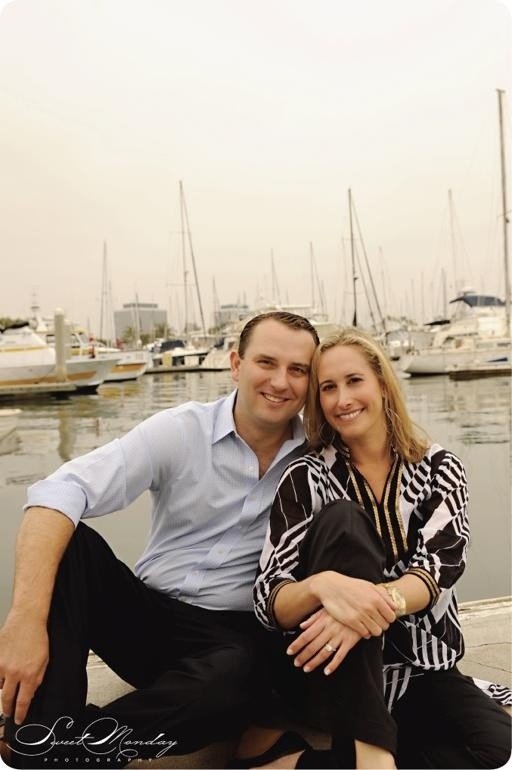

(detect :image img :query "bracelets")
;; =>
[381,581,407,618]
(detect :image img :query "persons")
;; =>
[251,324,512,769]
[0,308,309,769]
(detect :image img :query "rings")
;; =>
[324,643,337,653]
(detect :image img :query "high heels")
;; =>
[224,726,311,769]
